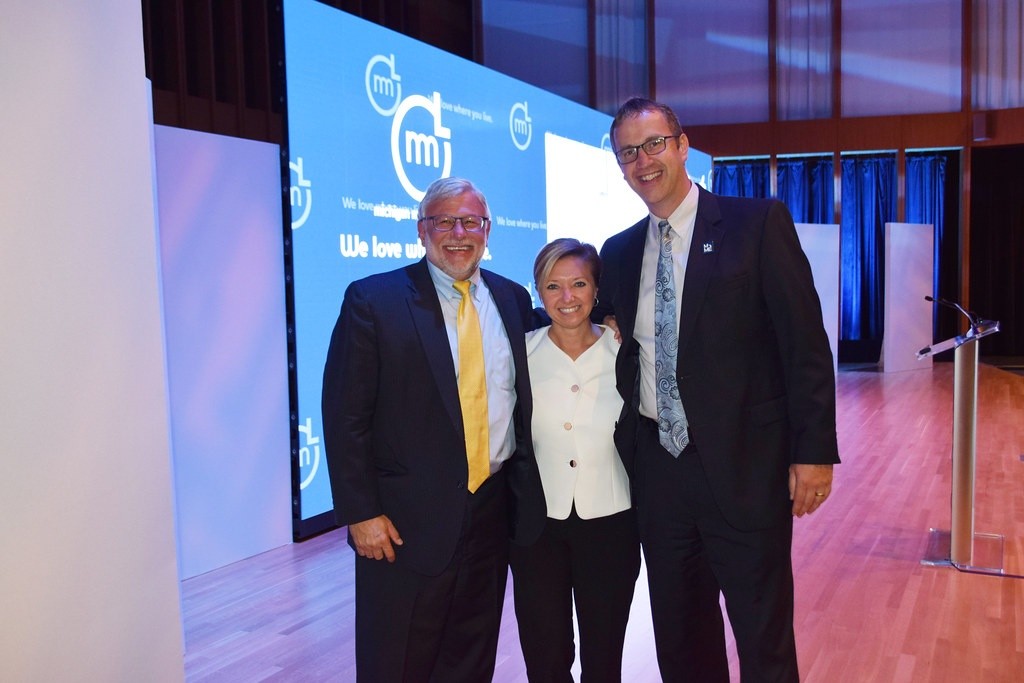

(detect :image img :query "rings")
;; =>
[817,493,824,496]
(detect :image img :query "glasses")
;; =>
[615,135,680,164]
[417,214,489,231]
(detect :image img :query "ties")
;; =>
[451,280,490,494]
[655,221,690,458]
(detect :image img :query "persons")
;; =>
[509,238,645,683]
[598,97,841,682]
[320,178,622,683]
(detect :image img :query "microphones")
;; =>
[943,297,974,327]
[925,295,979,322]
